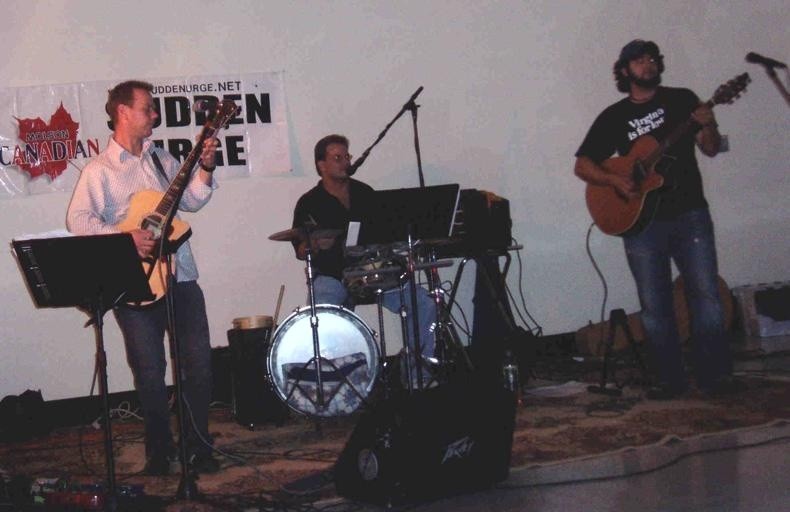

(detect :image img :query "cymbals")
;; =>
[268,224,343,242]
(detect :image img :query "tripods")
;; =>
[284,249,366,417]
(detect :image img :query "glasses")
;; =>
[329,153,353,161]
[634,54,657,64]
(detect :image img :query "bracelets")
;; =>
[198,159,217,173]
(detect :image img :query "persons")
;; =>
[66,80,222,477]
[286,134,442,393]
[573,39,744,400]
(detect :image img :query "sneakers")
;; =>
[140,438,221,479]
[644,369,750,398]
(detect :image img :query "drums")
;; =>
[264,304,380,419]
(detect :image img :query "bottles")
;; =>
[502,350,519,393]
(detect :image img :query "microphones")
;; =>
[744,52,786,69]
[192,99,219,112]
[347,157,364,176]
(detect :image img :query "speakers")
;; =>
[332,380,518,509]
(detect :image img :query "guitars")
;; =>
[115,99,240,311]
[585,73,751,237]
